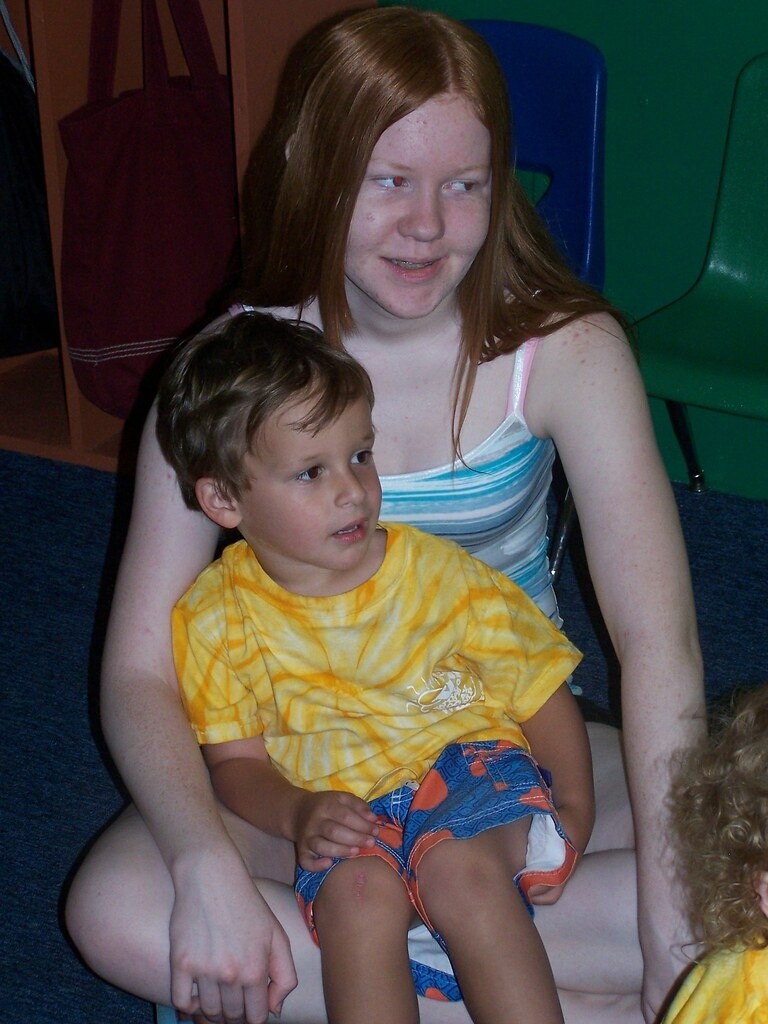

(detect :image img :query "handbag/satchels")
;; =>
[60,2,242,420]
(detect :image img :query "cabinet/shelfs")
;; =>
[0,0,378,480]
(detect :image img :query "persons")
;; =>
[67,4,723,1024]
[153,308,596,1024]
[656,690,768,1024]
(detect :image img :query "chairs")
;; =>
[608,51,767,501]
[432,18,615,324]
